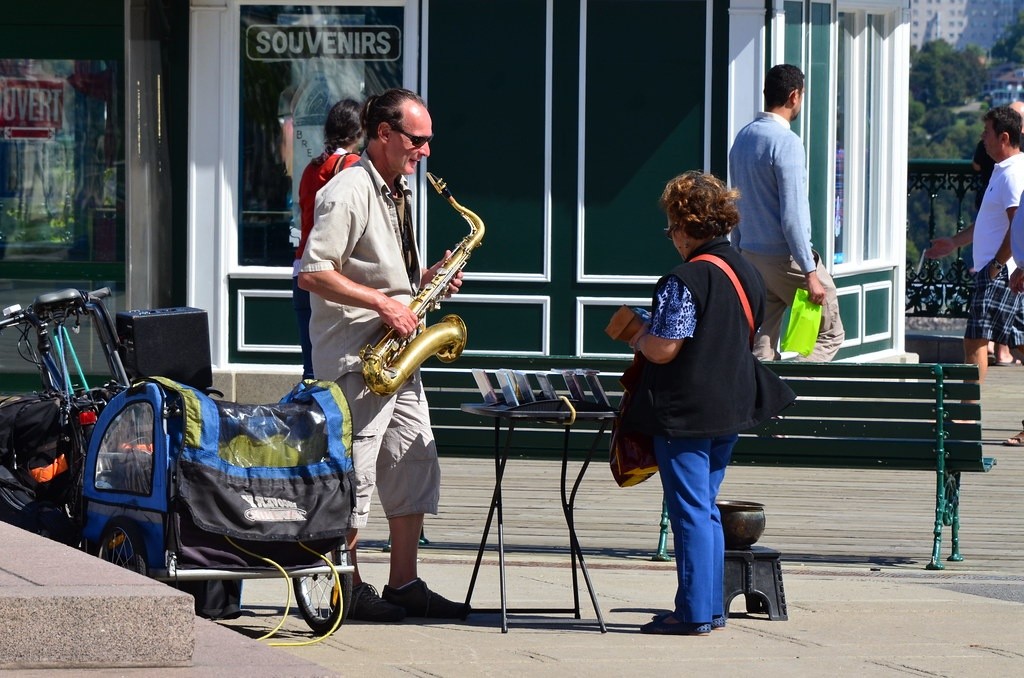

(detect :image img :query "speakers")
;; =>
[117,306,213,390]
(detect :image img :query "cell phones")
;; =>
[562,372,587,402]
[535,371,559,400]
[496,367,537,407]
[583,373,611,407]
[471,369,498,403]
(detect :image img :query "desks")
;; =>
[458,400,614,633]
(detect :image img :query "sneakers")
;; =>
[383,579,471,618]
[328,582,407,622]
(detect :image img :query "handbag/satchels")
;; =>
[609,347,662,487]
[774,287,822,362]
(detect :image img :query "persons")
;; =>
[729,65,845,363]
[627,170,797,636]
[924,101,1024,447]
[293,99,363,381]
[297,88,473,625]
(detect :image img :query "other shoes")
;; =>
[641,617,711,636]
[654,613,726,630]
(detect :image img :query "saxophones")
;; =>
[359,172,486,396]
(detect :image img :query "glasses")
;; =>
[662,220,683,237]
[392,129,436,145]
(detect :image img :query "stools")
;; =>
[675,544,789,622]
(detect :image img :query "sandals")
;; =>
[1003,431,1024,446]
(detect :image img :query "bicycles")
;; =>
[0,285,114,517]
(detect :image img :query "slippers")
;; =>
[987,353,1022,367]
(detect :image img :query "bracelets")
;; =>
[993,259,1004,273]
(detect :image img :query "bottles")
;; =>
[290,56,343,231]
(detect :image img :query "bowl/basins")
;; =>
[714,499,766,550]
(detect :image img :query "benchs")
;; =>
[420,354,999,570]
